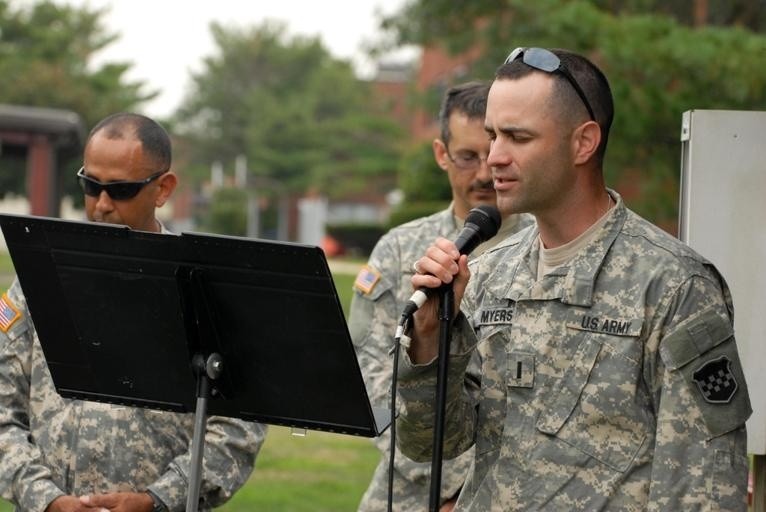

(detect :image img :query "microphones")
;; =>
[401,205,502,316]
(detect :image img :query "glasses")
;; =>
[76,159,172,199]
[504,42,601,128]
[441,141,492,172]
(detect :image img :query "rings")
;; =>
[410,259,426,275]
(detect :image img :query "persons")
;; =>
[347,79,538,512]
[387,44,755,511]
[0,110,269,512]
